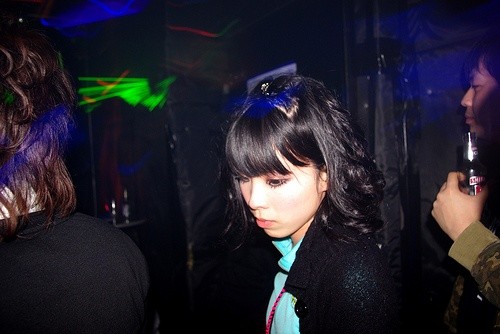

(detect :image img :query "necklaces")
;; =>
[265,286,286,334]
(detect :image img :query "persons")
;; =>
[0,22,161,334]
[431,43,500,334]
[225,73,393,334]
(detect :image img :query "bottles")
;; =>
[460,132,485,195]
